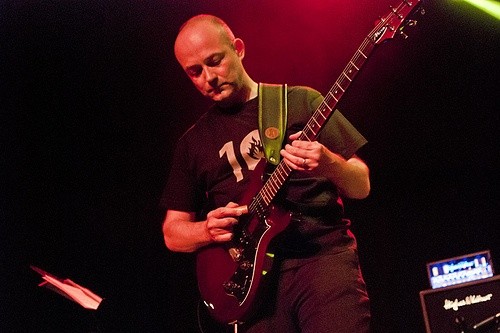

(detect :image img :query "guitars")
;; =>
[196,0,425,326]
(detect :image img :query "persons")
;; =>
[159,13,370,333]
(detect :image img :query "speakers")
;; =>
[419,274,500,333]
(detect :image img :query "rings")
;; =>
[302,159,306,168]
